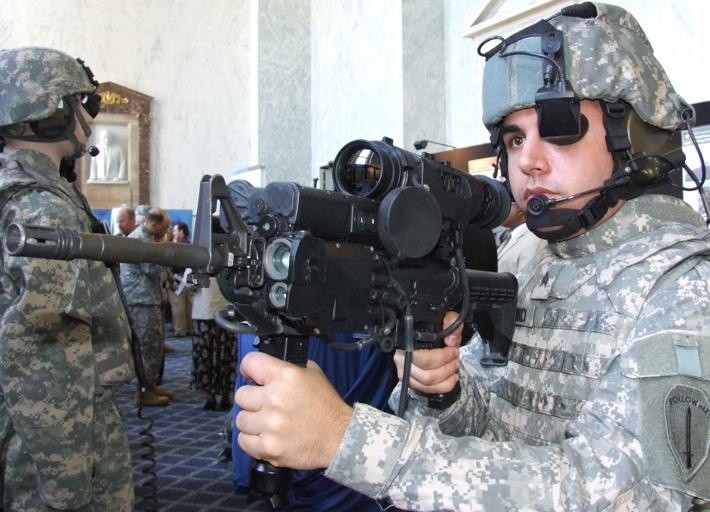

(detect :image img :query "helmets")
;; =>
[481,1,695,144]
[0,48,95,124]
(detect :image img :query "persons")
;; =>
[167,222,191,338]
[234,1,710,512]
[119,206,173,406]
[111,208,138,283]
[134,205,152,227]
[0,46,135,512]
[187,215,234,412]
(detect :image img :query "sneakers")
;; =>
[137,390,168,407]
[153,386,173,398]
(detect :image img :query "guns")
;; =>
[0,138,519,501]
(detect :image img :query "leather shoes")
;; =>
[216,395,232,410]
[193,394,216,411]
